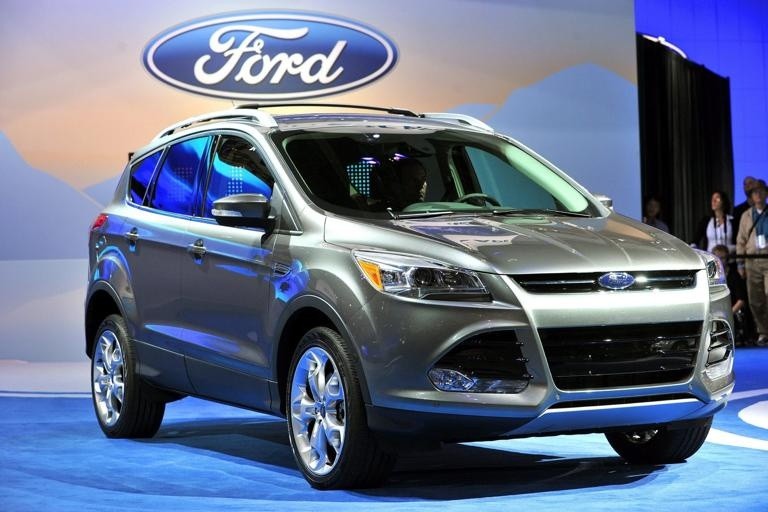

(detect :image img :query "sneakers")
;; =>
[756,334,767,345]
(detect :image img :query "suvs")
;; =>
[82,101,736,493]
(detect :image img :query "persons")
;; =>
[699,192,740,263]
[730,176,757,246]
[637,195,675,233]
[369,152,431,213]
[700,240,749,337]
[744,176,768,343]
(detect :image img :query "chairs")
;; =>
[316,160,399,208]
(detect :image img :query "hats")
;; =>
[745,180,768,197]
